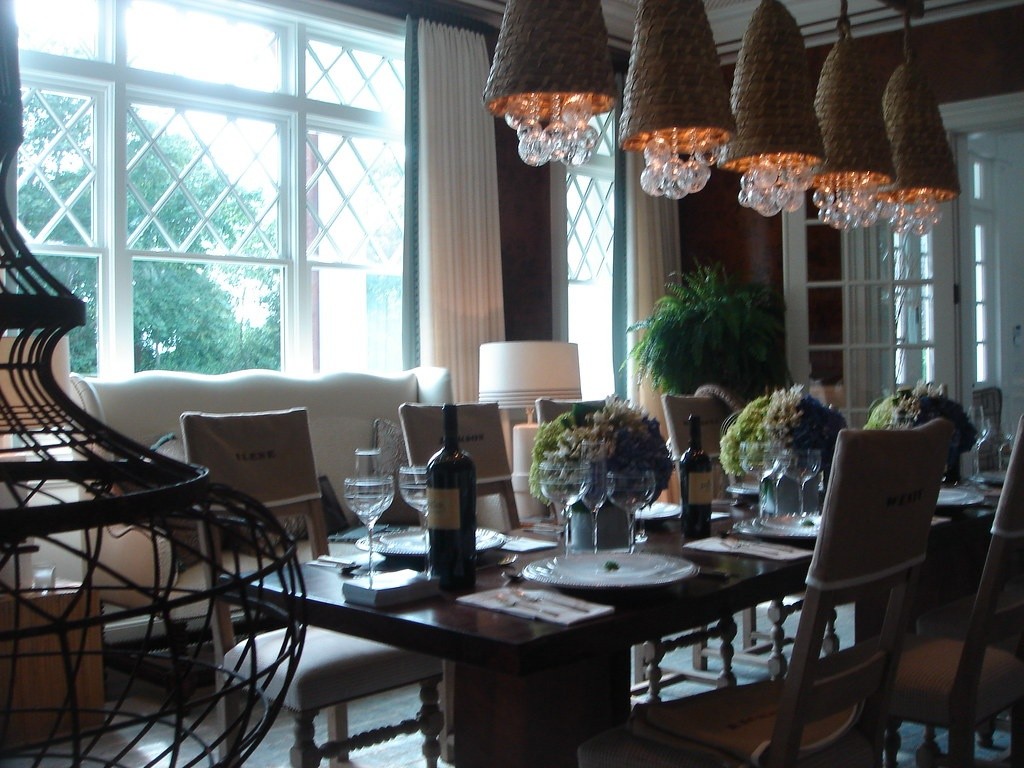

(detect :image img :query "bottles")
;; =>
[426,405,477,591]
[679,415,712,538]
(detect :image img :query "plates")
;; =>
[733,515,822,537]
[636,502,682,519]
[356,528,507,564]
[522,551,700,587]
[936,489,983,505]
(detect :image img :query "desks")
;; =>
[210,477,997,768]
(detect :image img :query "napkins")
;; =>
[340,567,441,608]
[454,581,615,627]
[682,535,813,564]
[500,535,559,553]
[929,514,953,527]
[676,511,731,522]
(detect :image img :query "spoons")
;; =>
[497,593,558,617]
[513,591,588,613]
[305,561,362,572]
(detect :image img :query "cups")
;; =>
[33,562,54,595]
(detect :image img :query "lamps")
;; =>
[477,341,584,493]
[479,0,961,237]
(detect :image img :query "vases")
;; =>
[757,474,820,515]
[569,505,634,551]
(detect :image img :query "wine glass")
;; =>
[783,450,822,526]
[345,448,395,588]
[986,416,1014,478]
[740,442,781,527]
[540,461,591,566]
[967,406,990,483]
[607,472,656,563]
[581,441,607,571]
[768,448,792,527]
[399,467,439,583]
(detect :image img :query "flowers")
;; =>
[862,378,979,455]
[528,392,676,512]
[719,383,849,501]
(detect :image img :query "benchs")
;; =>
[30,365,456,718]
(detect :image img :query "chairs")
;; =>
[178,407,446,768]
[536,393,738,703]
[816,413,1024,768]
[577,415,949,768]
[397,401,521,530]
[659,392,840,682]
